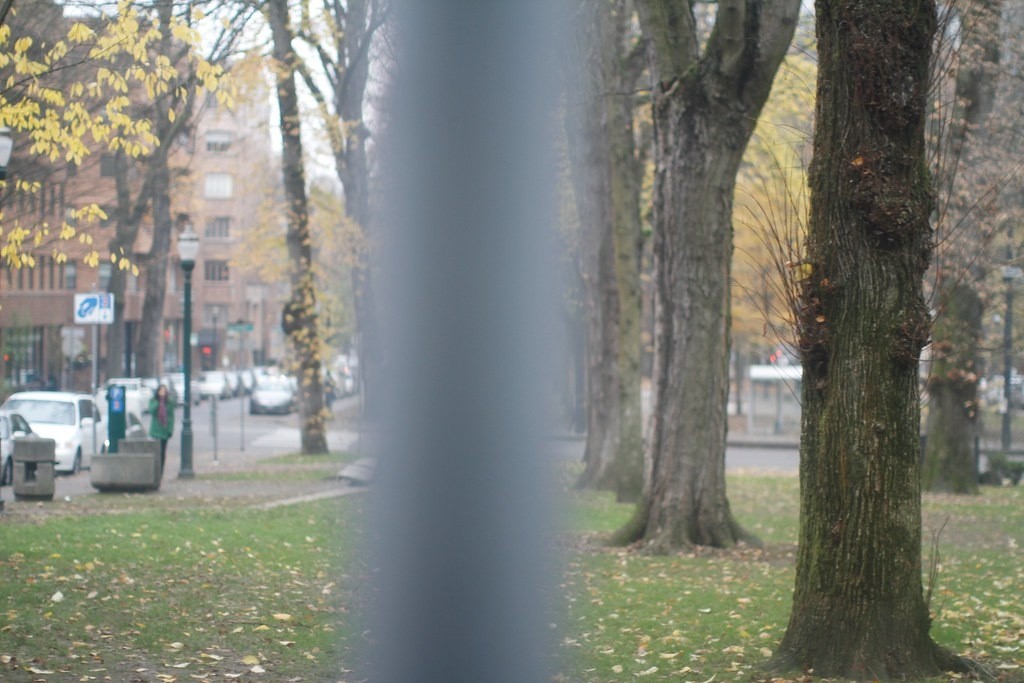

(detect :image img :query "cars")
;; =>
[248,375,293,415]
[320,355,361,401]
[0,411,41,486]
[90,367,278,433]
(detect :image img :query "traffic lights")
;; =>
[201,343,213,372]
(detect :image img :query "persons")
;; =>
[149,385,175,476]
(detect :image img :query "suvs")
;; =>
[2,390,107,477]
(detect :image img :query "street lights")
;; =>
[173,223,200,481]
